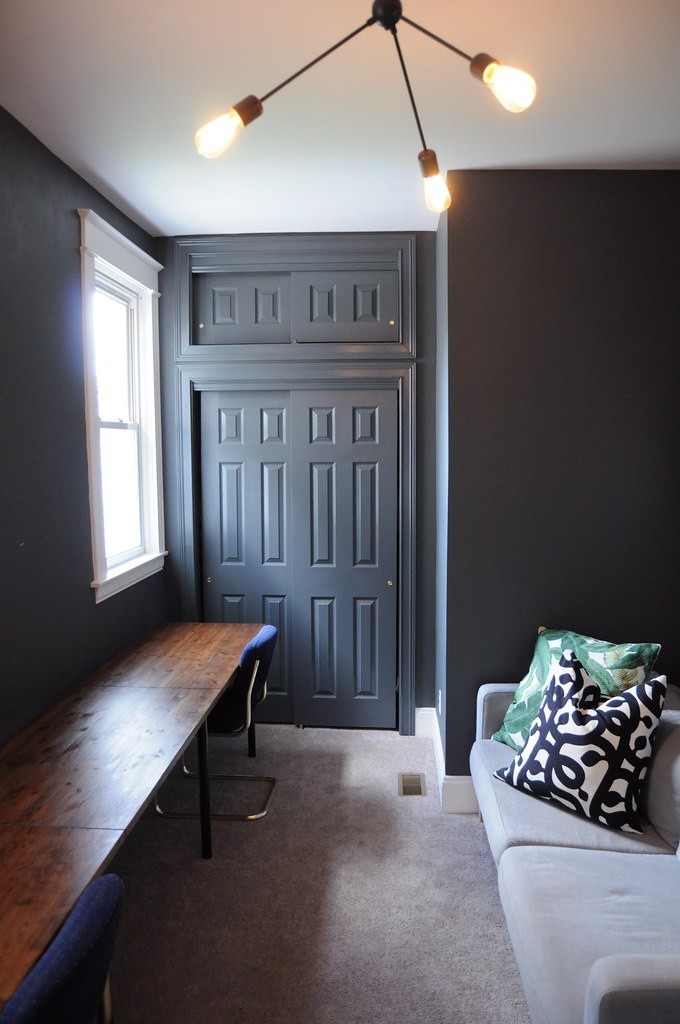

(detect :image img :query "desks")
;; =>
[0,621,268,1024]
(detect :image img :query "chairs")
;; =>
[0,871,129,1024]
[154,624,279,823]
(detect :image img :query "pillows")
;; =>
[490,627,662,755]
[492,649,668,836]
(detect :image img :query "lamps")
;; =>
[192,0,539,214]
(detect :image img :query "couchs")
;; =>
[469,682,680,1024]
